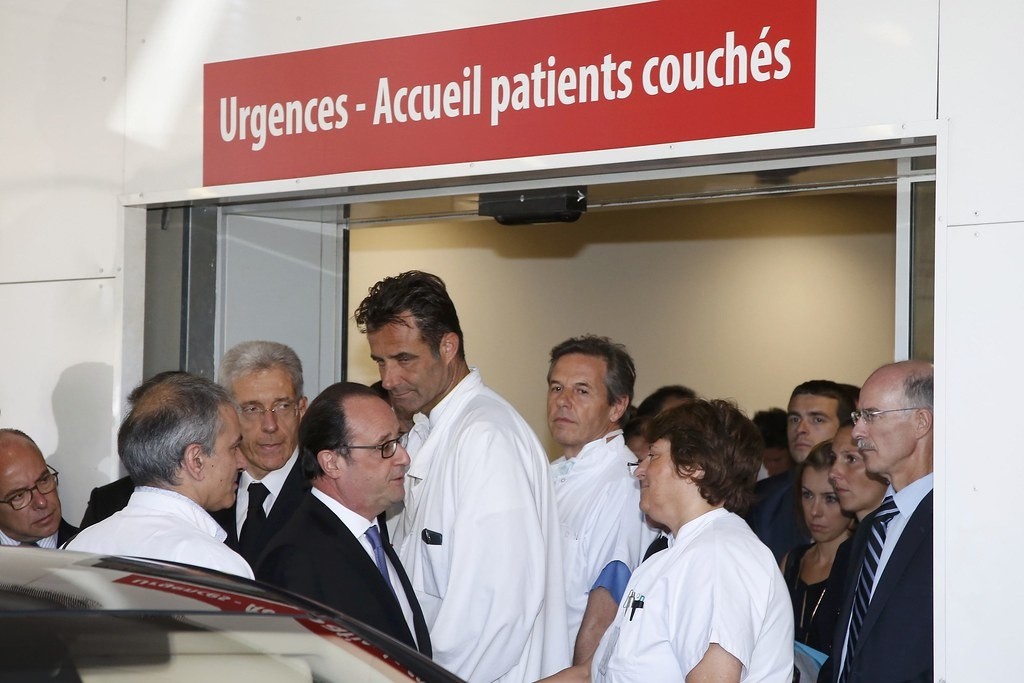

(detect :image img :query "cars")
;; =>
[0,545,470,683]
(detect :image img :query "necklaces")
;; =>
[797,547,830,643]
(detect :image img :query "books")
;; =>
[793,640,830,683]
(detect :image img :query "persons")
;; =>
[821,394,890,683]
[538,399,795,683]
[210,339,308,572]
[740,379,857,611]
[787,438,859,682]
[367,380,415,562]
[830,355,933,683]
[546,330,662,683]
[612,380,868,482]
[80,371,193,531]
[355,270,567,682]
[255,383,433,661]
[57,374,256,582]
[0,428,84,551]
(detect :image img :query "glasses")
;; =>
[850,407,920,426]
[238,400,299,422]
[332,430,409,458]
[0,464,58,510]
[627,461,642,479]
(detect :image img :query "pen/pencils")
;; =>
[622,589,644,621]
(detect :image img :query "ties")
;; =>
[238,481,271,562]
[363,525,401,610]
[838,494,900,683]
